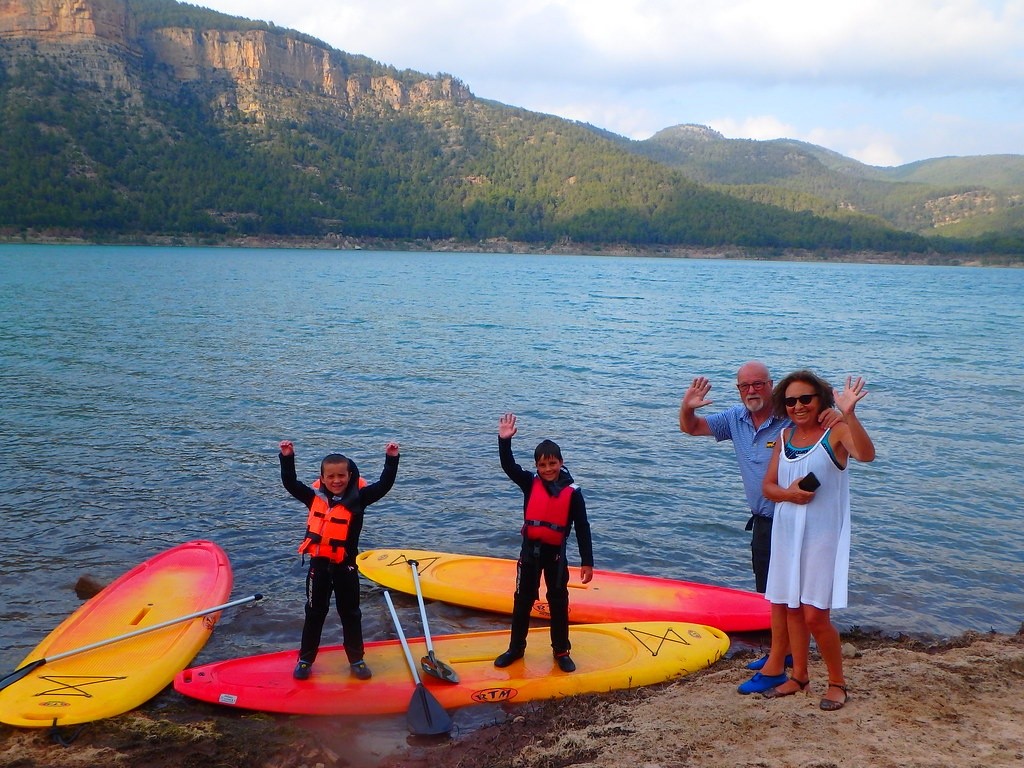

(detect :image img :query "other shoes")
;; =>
[350,660,372,680]
[747,652,793,669]
[494,649,524,668]
[293,660,312,678]
[737,671,788,694]
[552,648,576,673]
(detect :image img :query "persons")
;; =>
[493,412,594,673]
[763,370,875,711]
[679,362,847,694]
[278,439,401,680]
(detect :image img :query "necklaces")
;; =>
[800,429,817,440]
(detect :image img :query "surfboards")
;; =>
[355,545,782,633]
[0,538,235,730]
[175,620,737,719]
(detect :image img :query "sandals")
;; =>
[819,684,847,711]
[762,677,810,699]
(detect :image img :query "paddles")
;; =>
[407,558,458,681]
[377,589,455,738]
[0,595,267,690]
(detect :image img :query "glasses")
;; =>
[784,393,819,407]
[737,380,773,392]
